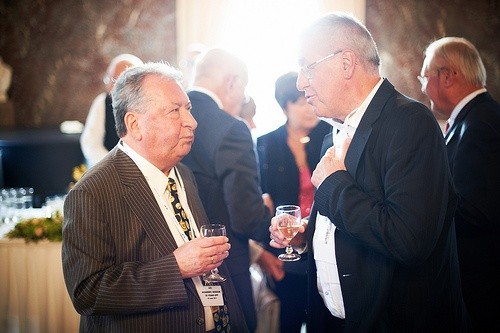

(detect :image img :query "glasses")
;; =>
[302,50,342,78]
[417,69,457,84]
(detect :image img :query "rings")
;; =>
[210,255,213,264]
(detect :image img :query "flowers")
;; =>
[4,210,63,243]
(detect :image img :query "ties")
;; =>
[445,121,449,132]
[165,177,231,333]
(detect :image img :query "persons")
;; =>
[269,15,448,332]
[173,48,273,332]
[78,53,145,167]
[239,94,258,130]
[62,65,231,333]
[256,71,342,333]
[417,36,499,332]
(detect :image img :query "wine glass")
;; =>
[275,204,301,261]
[200,224,227,282]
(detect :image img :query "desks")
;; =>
[0,232,81,333]
